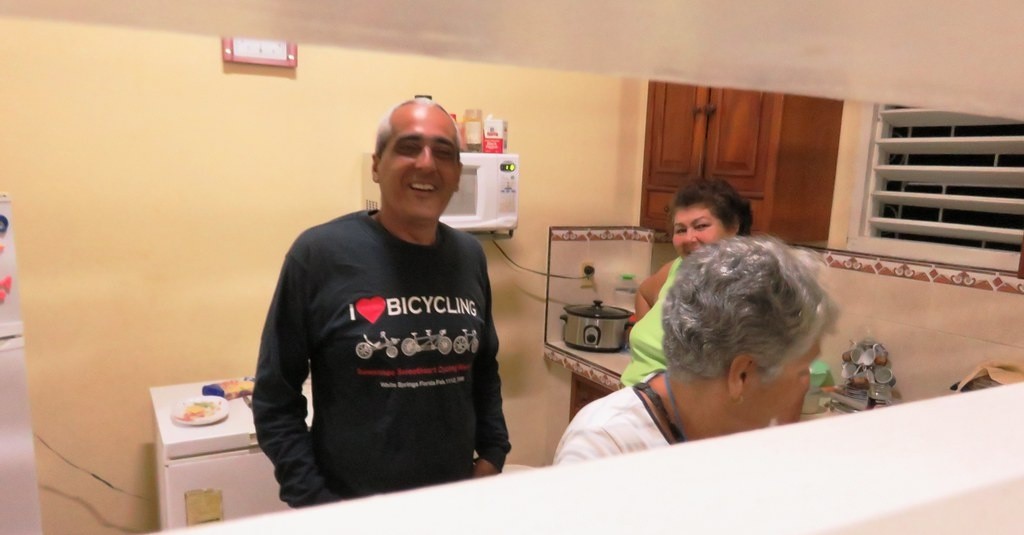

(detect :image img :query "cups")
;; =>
[841,340,896,385]
[866,385,892,412]
[796,359,833,414]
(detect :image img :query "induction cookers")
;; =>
[362,151,521,237]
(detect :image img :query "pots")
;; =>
[559,300,634,352]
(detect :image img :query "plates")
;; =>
[170,395,230,425]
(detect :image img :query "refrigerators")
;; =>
[0,192,44,535]
[148,374,299,531]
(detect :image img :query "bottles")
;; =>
[462,109,483,151]
[614,274,638,313]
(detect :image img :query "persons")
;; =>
[620,177,753,386]
[252,98,512,510]
[553,235,840,467]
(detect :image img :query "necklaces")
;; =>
[634,370,689,443]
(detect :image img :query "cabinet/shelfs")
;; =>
[149,375,315,530]
[639,81,844,248]
